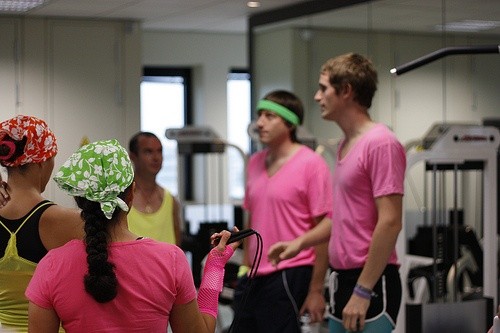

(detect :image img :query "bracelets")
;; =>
[353,284,373,299]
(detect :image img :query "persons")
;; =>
[125,132,180,248]
[266,52,406,333]
[25,139,242,333]
[0,115,86,333]
[228,91,332,333]
